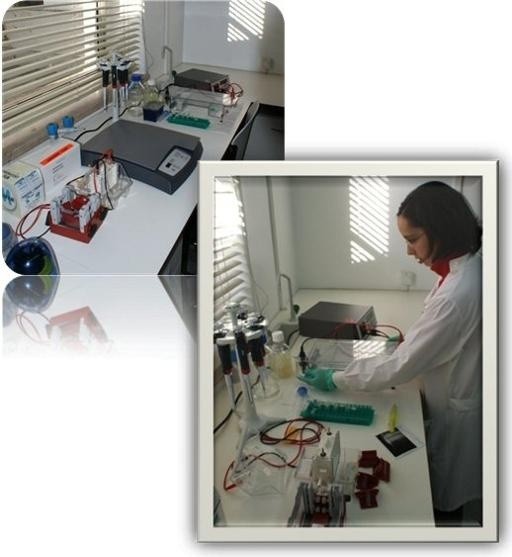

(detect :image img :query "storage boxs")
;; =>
[21,138,81,200]
[2,163,45,218]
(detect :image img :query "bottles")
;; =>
[266,329,295,382]
[127,72,160,117]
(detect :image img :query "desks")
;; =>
[214,288,436,527]
[8,61,284,275]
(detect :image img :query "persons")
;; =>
[294,180,482,526]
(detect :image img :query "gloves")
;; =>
[298,366,338,393]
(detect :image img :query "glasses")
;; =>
[406,232,427,244]
